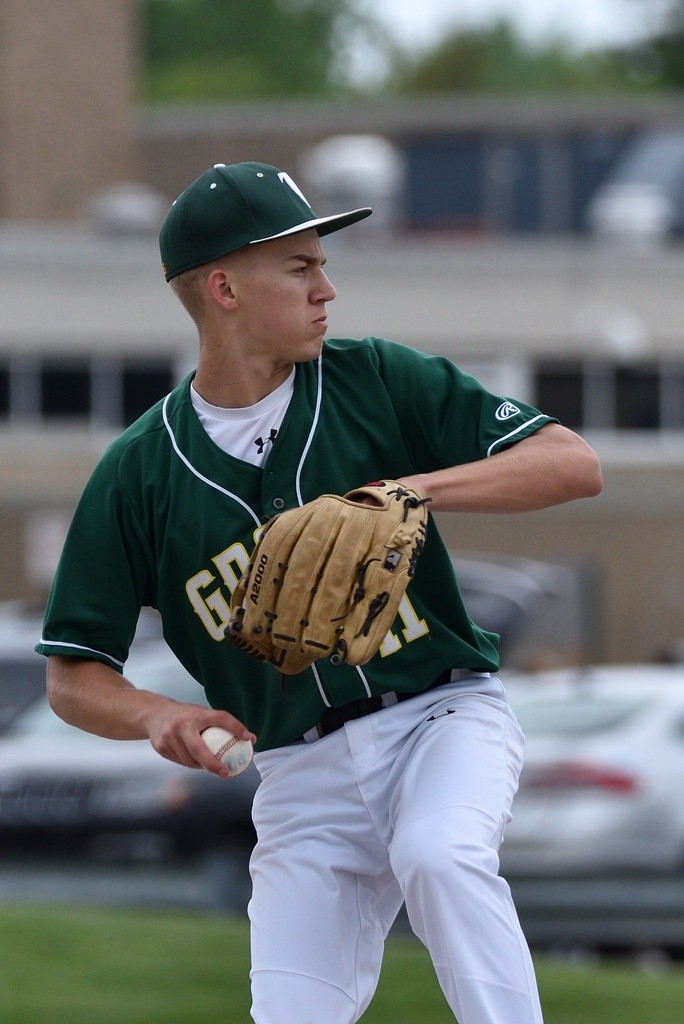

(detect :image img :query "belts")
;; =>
[281,669,453,745]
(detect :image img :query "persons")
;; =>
[33,161,604,1024]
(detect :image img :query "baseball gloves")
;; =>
[226,479,430,679]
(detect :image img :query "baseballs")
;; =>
[199,729,255,777]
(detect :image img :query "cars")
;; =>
[492,662,681,945]
[0,520,592,866]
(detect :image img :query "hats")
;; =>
[159,162,372,282]
